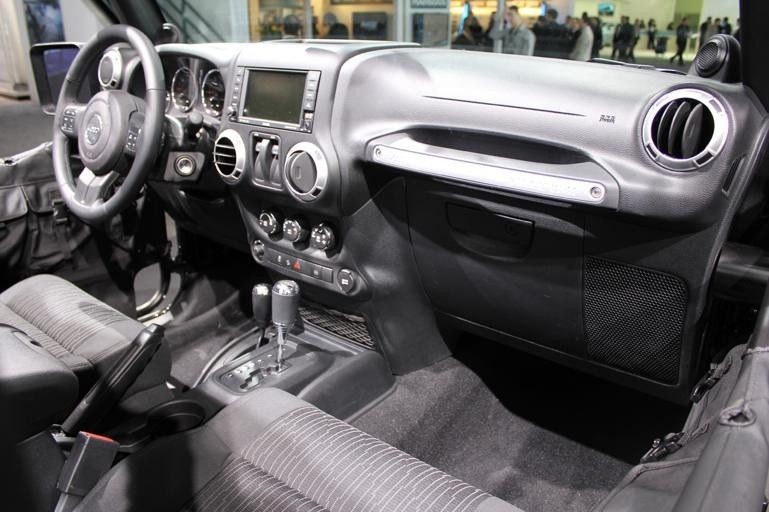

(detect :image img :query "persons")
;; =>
[279,7,349,39]
[454,0,740,65]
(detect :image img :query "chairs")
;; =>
[68,385,526,511]
[2,274,175,439]
